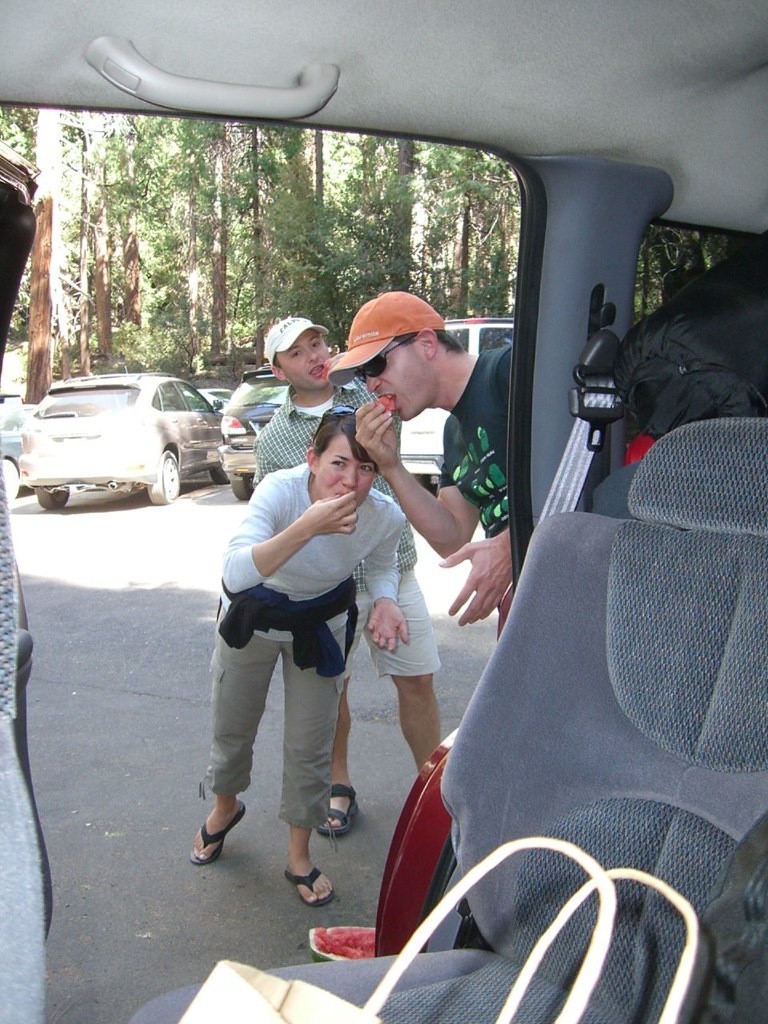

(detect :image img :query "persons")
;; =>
[323,289,516,643]
[252,316,442,837]
[183,404,409,907]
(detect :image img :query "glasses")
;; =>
[353,334,416,383]
[313,405,359,443]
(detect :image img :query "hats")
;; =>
[327,291,447,386]
[266,318,328,370]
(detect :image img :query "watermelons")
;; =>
[322,359,328,380]
[375,396,394,412]
[309,926,376,962]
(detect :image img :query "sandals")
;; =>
[316,785,358,835]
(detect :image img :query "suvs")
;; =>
[219,368,283,503]
[392,317,514,487]
[20,373,226,512]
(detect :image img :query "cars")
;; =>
[0,403,39,504]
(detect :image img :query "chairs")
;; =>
[129,416,768,1024]
[0,147,55,1024]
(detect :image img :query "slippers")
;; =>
[284,865,333,906]
[191,800,246,866]
